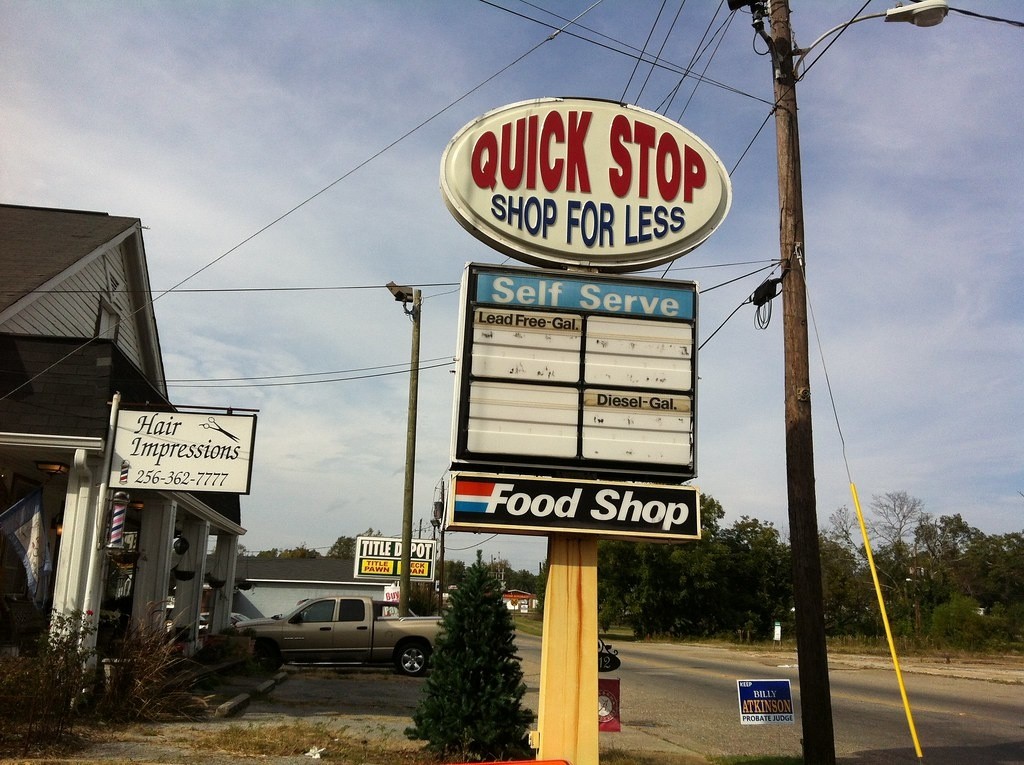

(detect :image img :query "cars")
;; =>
[165,602,208,635]
[201,611,250,624]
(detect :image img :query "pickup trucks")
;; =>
[231,594,447,677]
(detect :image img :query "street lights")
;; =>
[430,520,446,617]
[763,0,952,765]
[386,279,422,619]
[905,576,921,649]
